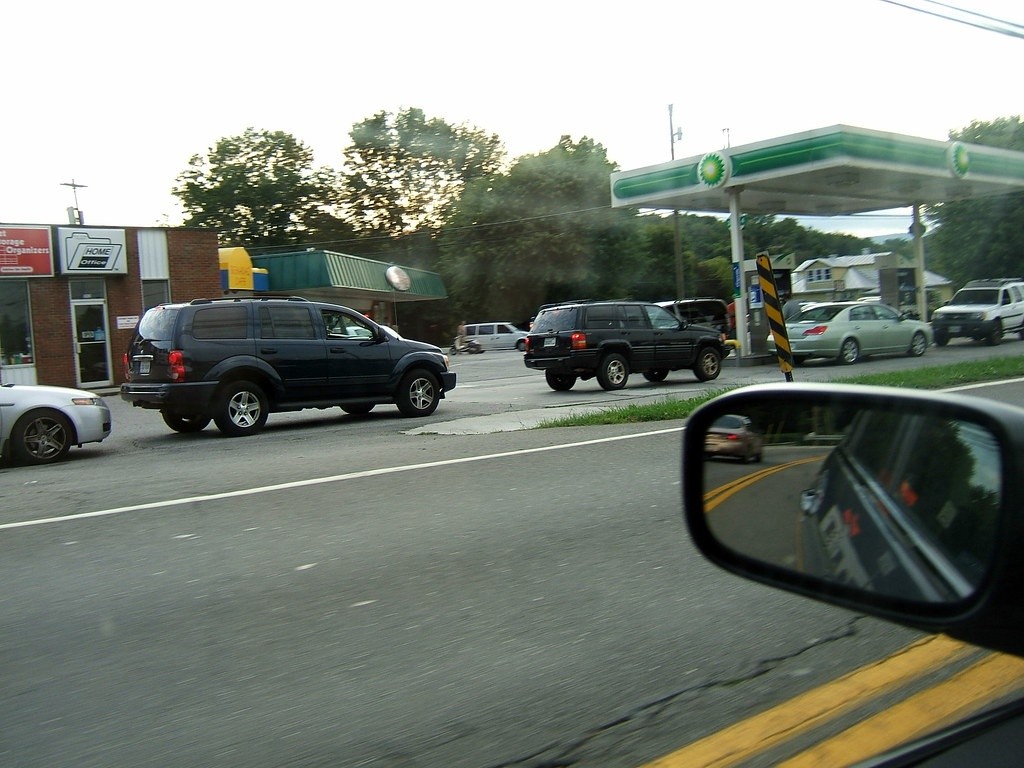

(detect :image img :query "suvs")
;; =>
[524,298,731,393]
[462,321,531,352]
[120,294,456,439]
[648,298,732,337]
[931,277,1024,348]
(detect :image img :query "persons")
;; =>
[457,321,466,346]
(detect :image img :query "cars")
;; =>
[783,302,935,366]
[0,381,112,467]
[702,415,765,464]
